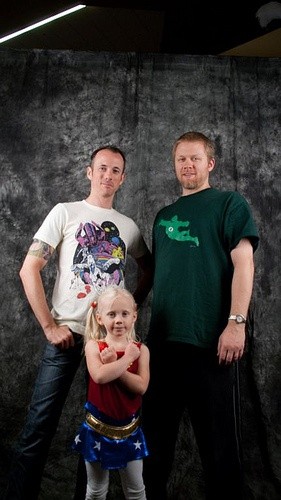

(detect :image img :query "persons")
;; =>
[8,144,158,500]
[150,131,259,500]
[72,285,152,500]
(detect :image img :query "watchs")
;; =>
[229,313,248,324]
